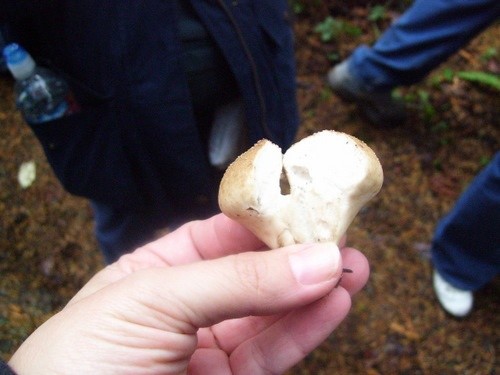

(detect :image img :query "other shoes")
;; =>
[328,60,406,128]
[432,268,474,318]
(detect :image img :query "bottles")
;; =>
[4,43,78,123]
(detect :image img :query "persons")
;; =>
[326,0,500,318]
[0,200,371,375]
